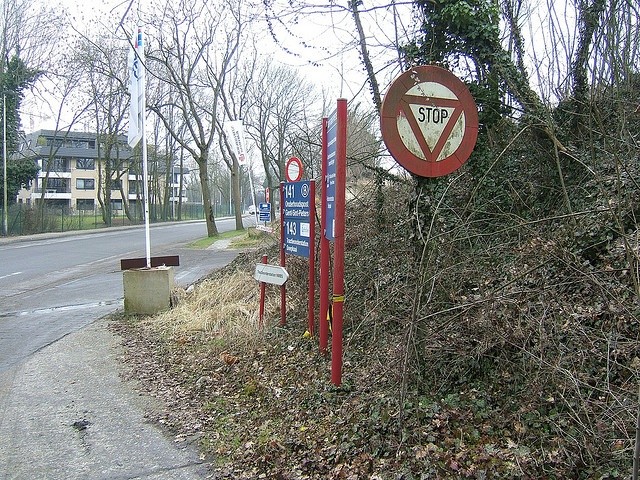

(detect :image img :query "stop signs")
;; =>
[379,65,479,177]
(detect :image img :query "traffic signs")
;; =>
[254,264,288,286]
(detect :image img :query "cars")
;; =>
[248,204,259,215]
